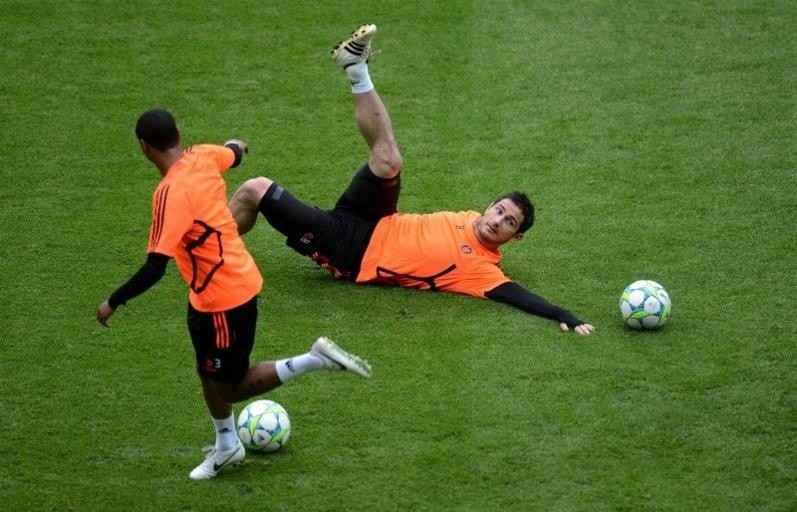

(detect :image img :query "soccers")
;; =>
[619,281,671,330]
[237,400,290,453]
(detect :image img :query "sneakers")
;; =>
[330,21,377,71]
[188,437,247,481]
[311,337,372,379]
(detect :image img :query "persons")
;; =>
[228,22,598,337]
[97,109,372,482]
